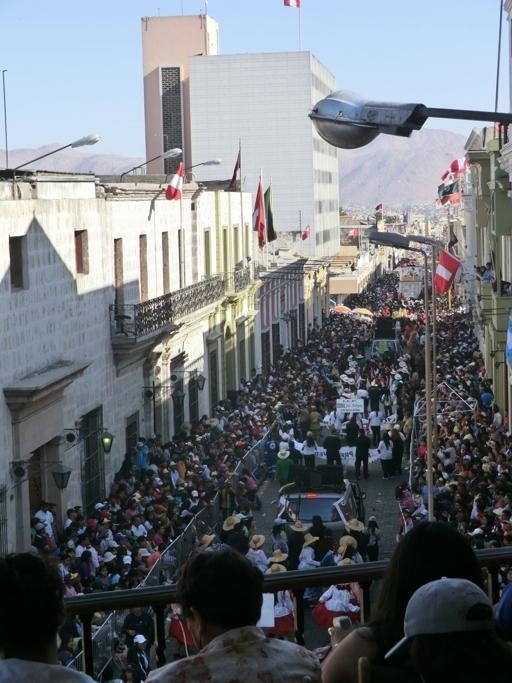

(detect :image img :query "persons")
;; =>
[0,551,95,681]
[28,254,512,683]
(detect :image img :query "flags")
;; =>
[432,157,465,207]
[282,0,301,11]
[248,166,270,250]
[164,160,184,203]
[263,185,278,241]
[223,138,244,192]
[432,231,461,295]
[347,225,363,236]
[374,202,383,211]
[300,221,312,241]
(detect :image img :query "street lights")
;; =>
[405,232,437,525]
[308,88,511,150]
[366,229,435,524]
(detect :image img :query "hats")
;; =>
[340,369,356,385]
[104,552,115,563]
[223,517,240,531]
[249,535,288,574]
[383,576,493,659]
[134,635,147,644]
[110,533,126,548]
[278,449,289,460]
[391,356,408,380]
[201,535,216,547]
[338,519,364,565]
[290,521,320,548]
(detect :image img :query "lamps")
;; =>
[119,143,184,183]
[305,79,511,151]
[63,424,115,457]
[10,130,102,185]
[142,384,186,412]
[183,158,221,171]
[5,452,73,493]
[170,368,206,391]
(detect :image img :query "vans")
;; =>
[370,338,403,362]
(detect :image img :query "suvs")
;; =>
[269,478,367,545]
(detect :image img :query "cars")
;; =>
[398,256,417,267]
